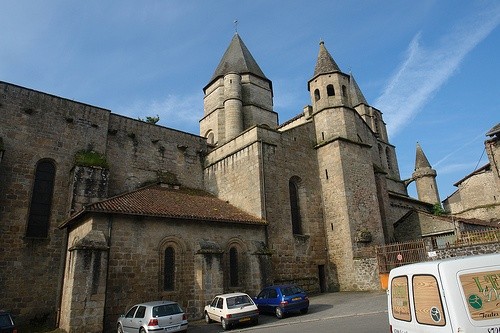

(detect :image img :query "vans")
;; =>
[388,254,500,333]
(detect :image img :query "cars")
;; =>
[0,307,18,333]
[117,300,189,333]
[253,284,310,319]
[204,292,259,330]
